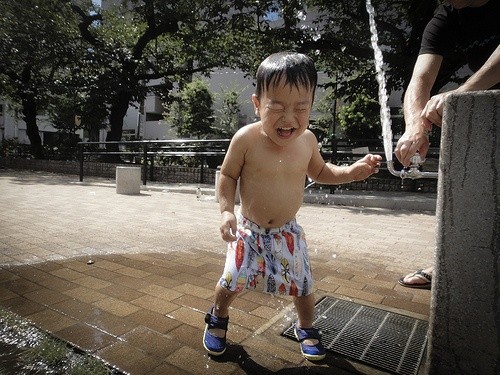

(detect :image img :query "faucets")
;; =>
[385,151,423,180]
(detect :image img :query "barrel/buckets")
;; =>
[115,162,142,195]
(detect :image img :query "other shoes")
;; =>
[294,325,326,361]
[203,305,229,356]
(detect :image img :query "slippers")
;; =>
[397,268,431,290]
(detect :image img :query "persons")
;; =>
[204,51,383,363]
[393,0,500,288]
